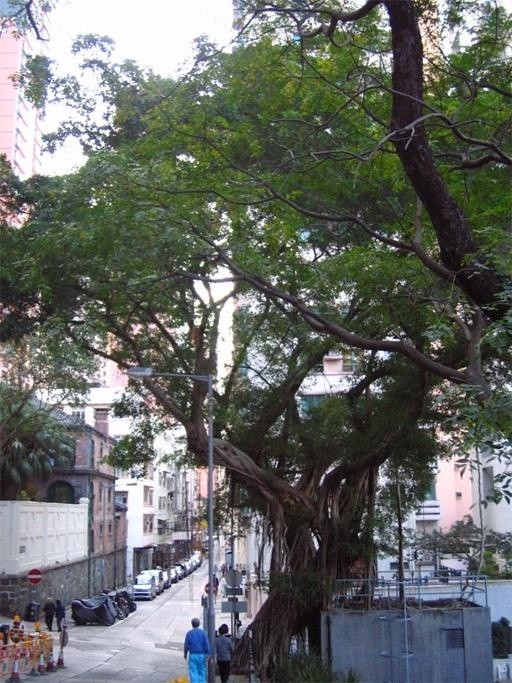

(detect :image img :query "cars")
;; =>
[131,550,202,601]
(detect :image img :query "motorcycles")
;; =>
[70,591,137,627]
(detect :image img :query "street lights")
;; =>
[128,366,216,682]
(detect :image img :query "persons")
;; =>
[183,617,235,683]
[43,596,64,632]
[213,573,219,595]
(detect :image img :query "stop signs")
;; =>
[204,582,218,595]
[26,569,42,586]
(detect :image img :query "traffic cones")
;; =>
[28,647,68,676]
[7,653,22,683]
